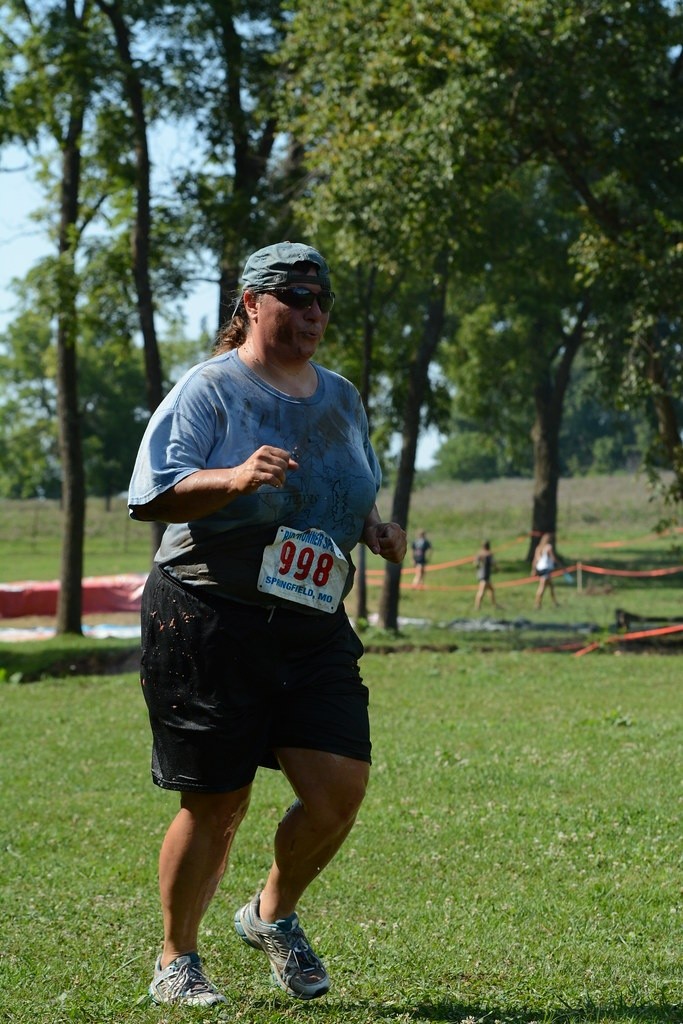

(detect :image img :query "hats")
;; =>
[231,240,331,319]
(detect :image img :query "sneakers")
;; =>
[234,894,331,1000]
[149,951,229,1008]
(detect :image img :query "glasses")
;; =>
[257,285,335,313]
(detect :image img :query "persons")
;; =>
[127,242,407,1009]
[411,528,432,586]
[531,532,562,610]
[474,540,502,612]
